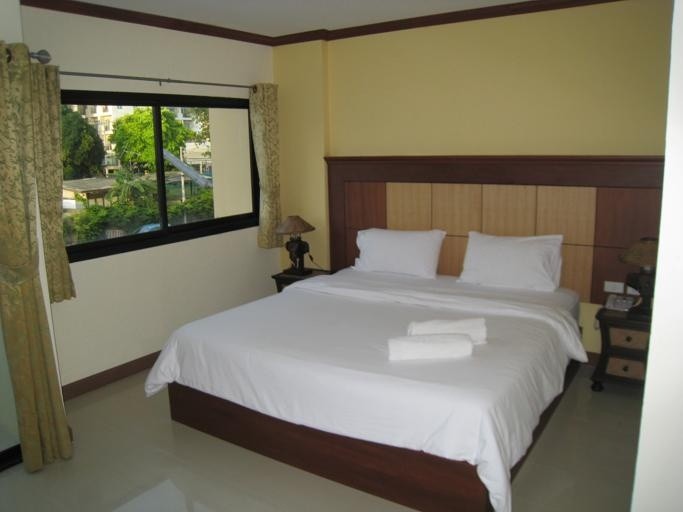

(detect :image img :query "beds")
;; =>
[167,266,582,511]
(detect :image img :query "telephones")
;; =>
[606,295,635,313]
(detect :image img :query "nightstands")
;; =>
[271,268,333,294]
[588,307,650,391]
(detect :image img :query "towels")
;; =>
[386,315,487,363]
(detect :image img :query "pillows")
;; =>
[350,227,565,294]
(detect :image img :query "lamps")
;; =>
[274,215,315,275]
[616,238,659,315]
